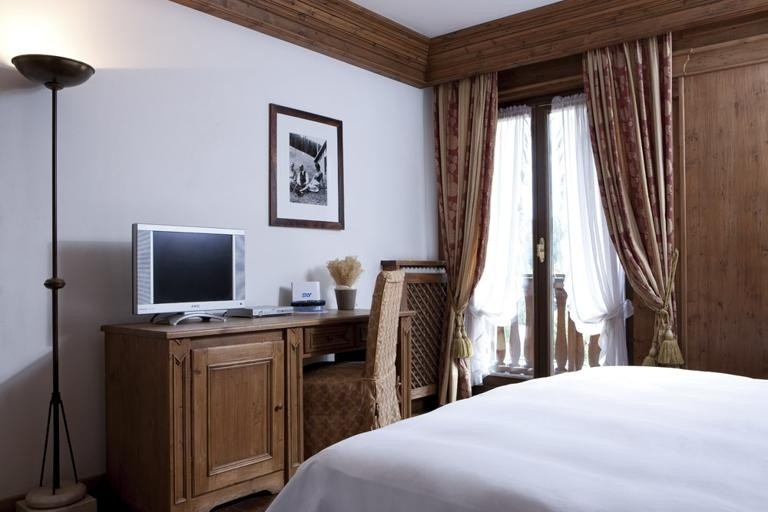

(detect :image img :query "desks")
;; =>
[99,310,468,512]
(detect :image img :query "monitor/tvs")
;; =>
[131,222,247,327]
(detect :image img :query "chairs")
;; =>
[303,271,407,465]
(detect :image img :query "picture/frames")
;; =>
[268,103,345,230]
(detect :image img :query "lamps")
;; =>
[12,53,101,512]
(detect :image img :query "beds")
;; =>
[287,360,768,512]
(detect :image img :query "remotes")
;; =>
[290,298,327,308]
